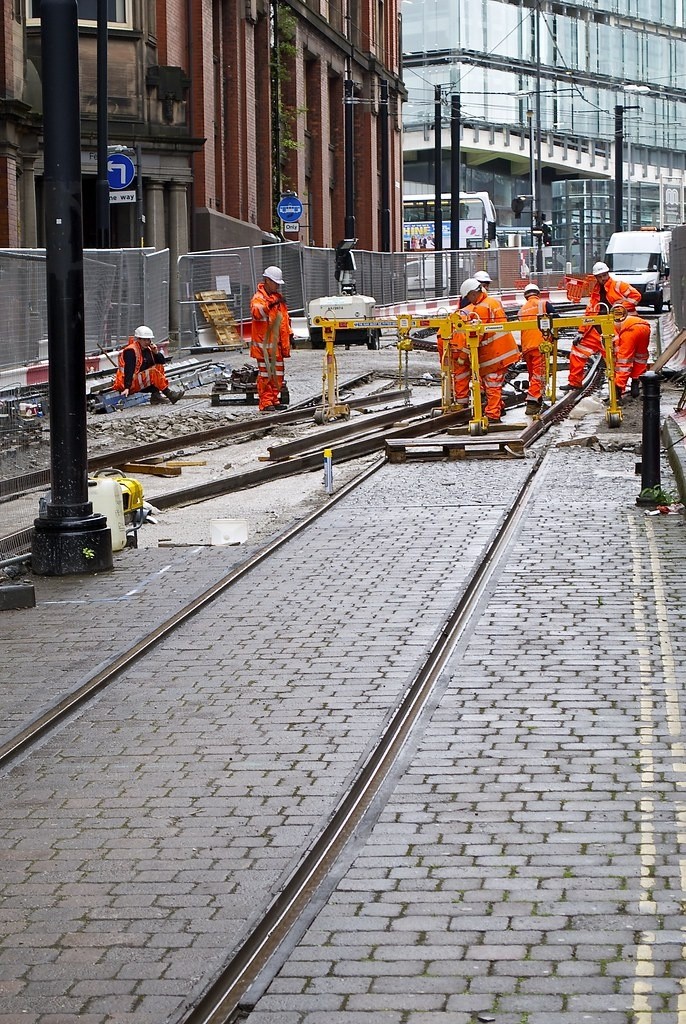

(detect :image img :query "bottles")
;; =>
[323,448,333,492]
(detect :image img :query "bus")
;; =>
[401,191,498,290]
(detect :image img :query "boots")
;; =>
[150,390,170,405]
[630,381,640,398]
[603,386,624,406]
[526,399,551,415]
[167,389,186,405]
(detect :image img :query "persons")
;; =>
[436,271,493,410]
[457,278,523,424]
[558,262,641,390]
[250,266,294,411]
[410,234,436,249]
[516,283,562,416]
[604,315,651,407]
[111,326,185,405]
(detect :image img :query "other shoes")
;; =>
[501,408,506,416]
[488,417,502,422]
[559,384,581,390]
[458,403,469,408]
[262,403,287,411]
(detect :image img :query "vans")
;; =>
[604,226,677,312]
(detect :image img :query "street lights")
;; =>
[622,84,650,231]
[514,88,536,271]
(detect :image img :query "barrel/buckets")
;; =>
[88,477,127,552]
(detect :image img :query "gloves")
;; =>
[289,334,296,349]
[573,333,583,346]
[267,295,279,309]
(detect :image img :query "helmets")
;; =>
[524,283,540,296]
[262,265,285,285]
[592,262,609,275]
[459,278,481,300]
[134,325,154,339]
[473,270,493,281]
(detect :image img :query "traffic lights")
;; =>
[544,227,551,246]
